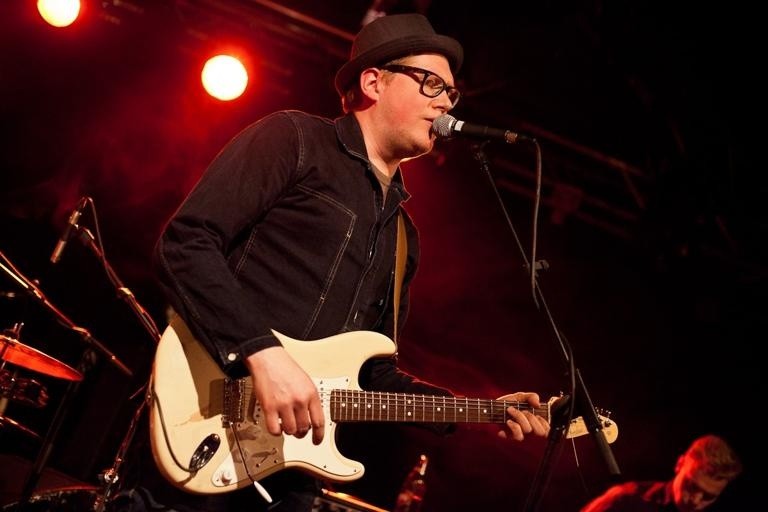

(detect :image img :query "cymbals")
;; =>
[0,335,83,384]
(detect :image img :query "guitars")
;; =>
[148,312,618,496]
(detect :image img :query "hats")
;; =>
[335,14,464,97]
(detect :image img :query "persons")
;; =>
[106,12,465,511]
[580,434,740,511]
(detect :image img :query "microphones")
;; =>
[50,196,91,265]
[432,114,520,159]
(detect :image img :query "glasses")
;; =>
[378,64,461,109]
